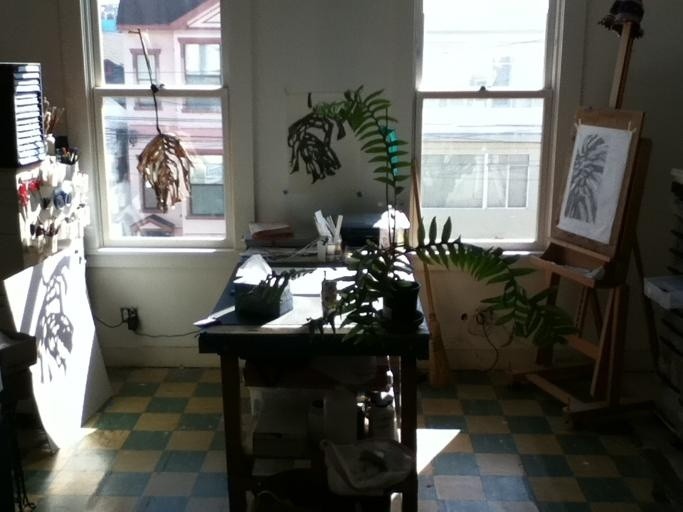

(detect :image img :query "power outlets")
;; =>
[121,307,138,323]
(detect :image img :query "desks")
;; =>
[198,247,432,512]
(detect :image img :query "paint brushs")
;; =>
[40,100,64,136]
[54,146,81,165]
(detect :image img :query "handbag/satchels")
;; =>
[320,436,413,495]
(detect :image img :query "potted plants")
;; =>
[287,85,579,348]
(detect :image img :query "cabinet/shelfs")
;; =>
[0,153,82,340]
